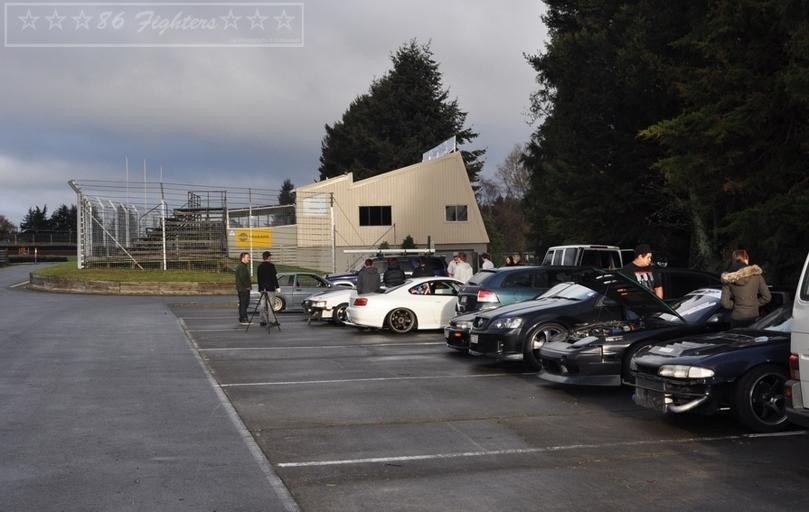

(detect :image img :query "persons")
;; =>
[454,252,473,283]
[720,249,772,329]
[481,252,529,270]
[447,251,460,278]
[257,251,280,327]
[383,257,405,288]
[235,252,253,325]
[623,243,664,300]
[412,258,435,278]
[356,258,381,295]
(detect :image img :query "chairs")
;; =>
[288,276,299,285]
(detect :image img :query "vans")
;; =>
[541,245,636,268]
[781,250,809,429]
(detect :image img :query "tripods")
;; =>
[242,296,285,336]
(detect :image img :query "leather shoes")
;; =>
[261,322,266,325]
[270,322,280,326]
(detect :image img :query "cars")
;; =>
[249,251,482,332]
[621,304,787,428]
